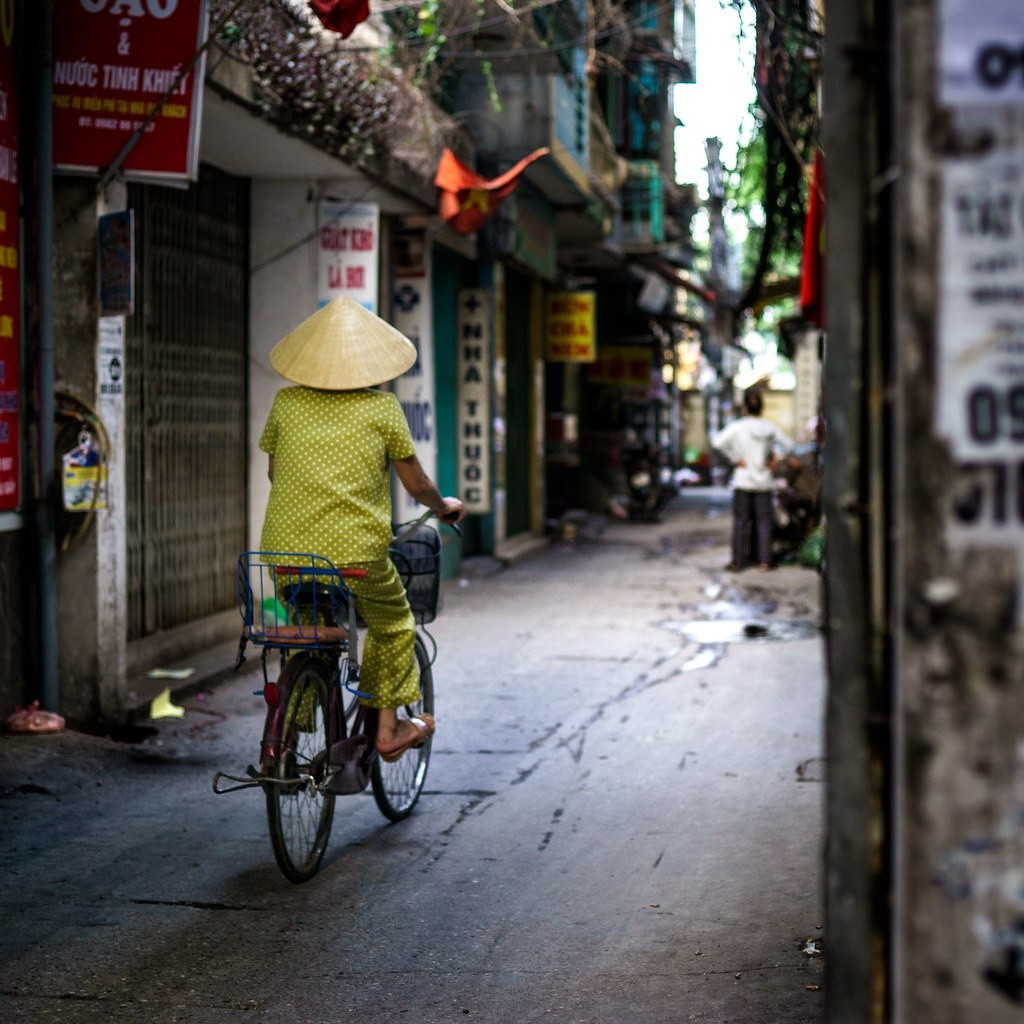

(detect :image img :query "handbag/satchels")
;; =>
[8,700,65,733]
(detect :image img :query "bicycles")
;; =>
[211,504,466,886]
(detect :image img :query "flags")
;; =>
[434,148,548,232]
[797,148,830,328]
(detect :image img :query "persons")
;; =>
[260,295,465,795]
[565,424,670,523]
[713,389,795,571]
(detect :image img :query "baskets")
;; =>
[353,523,442,628]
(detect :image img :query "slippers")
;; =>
[380,712,435,761]
[278,751,301,795]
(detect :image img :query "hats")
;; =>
[270,295,418,390]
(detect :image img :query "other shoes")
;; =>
[759,562,770,574]
